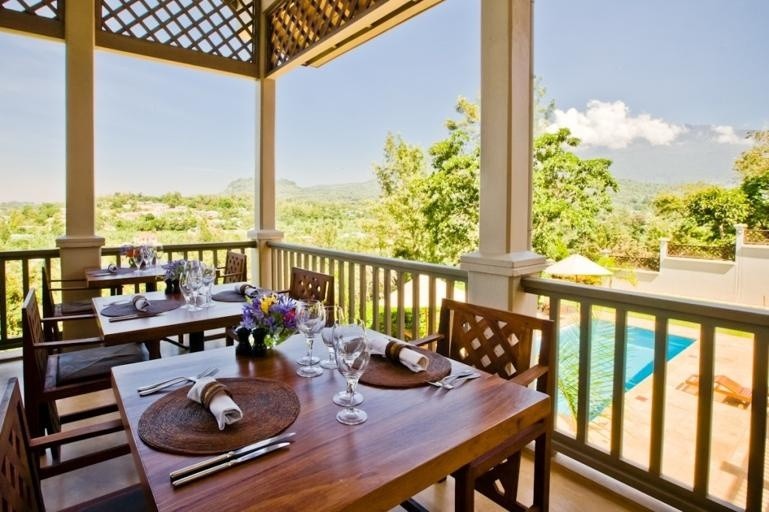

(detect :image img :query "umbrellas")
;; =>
[544,253,612,283]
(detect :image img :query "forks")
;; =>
[137,367,218,398]
[422,368,481,391]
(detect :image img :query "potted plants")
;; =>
[164,260,194,276]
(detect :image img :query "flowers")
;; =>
[244,287,302,346]
[121,243,148,257]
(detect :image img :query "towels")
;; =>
[107,262,120,275]
[185,373,243,430]
[235,277,258,298]
[128,289,154,314]
[364,331,427,375]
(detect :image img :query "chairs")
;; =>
[222,266,337,346]
[177,248,247,346]
[15,290,146,459]
[1,373,153,512]
[683,371,752,408]
[37,264,125,354]
[398,298,556,512]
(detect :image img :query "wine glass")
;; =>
[134,241,164,274]
[295,300,369,428]
[178,260,216,310]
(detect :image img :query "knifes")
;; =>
[172,442,290,488]
[170,431,296,480]
